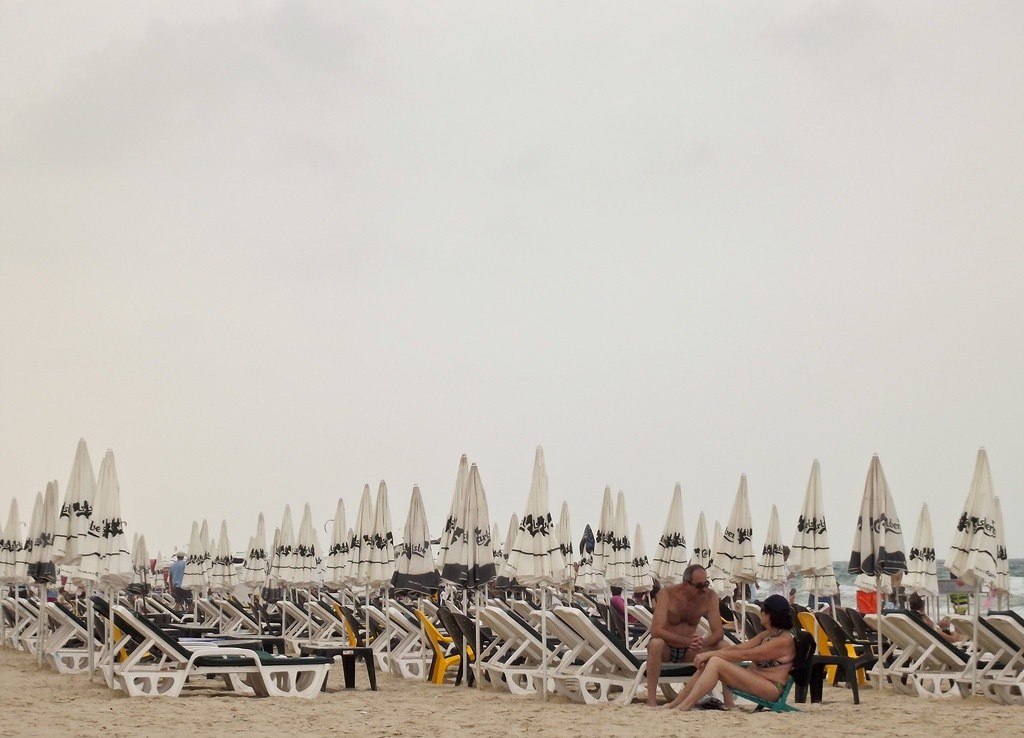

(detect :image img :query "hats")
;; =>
[177,551,185,557]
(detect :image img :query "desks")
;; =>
[143,613,172,623]
[205,634,285,679]
[296,645,378,693]
[168,623,218,639]
[794,655,860,705]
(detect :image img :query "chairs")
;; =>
[1,584,1024,712]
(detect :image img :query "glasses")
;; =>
[760,607,765,612]
[684,578,710,589]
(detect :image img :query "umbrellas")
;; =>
[154,444,1010,702]
[0,437,153,692]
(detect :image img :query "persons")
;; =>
[610,585,643,623]
[663,593,796,713]
[647,564,737,709]
[57,587,75,614]
[650,577,661,609]
[168,552,194,612]
[949,572,975,617]
[8,585,28,598]
[909,591,970,643]
